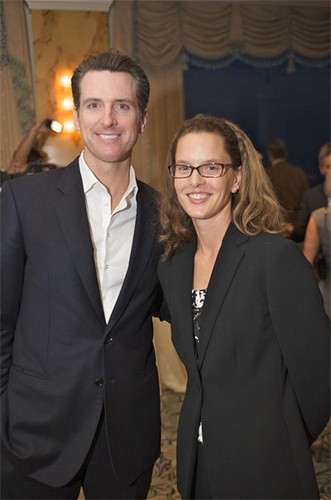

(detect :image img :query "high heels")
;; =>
[311,440,323,464]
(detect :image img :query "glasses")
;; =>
[168,163,237,178]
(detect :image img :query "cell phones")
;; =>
[46,119,62,133]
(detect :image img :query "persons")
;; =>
[2,120,54,184]
[253,136,331,321]
[158,115,331,500]
[0,50,171,500]
